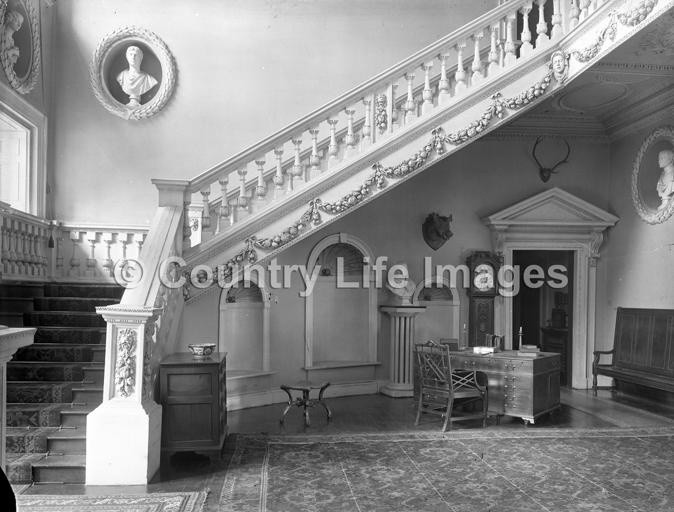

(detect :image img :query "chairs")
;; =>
[441,342,458,351]
[413,339,488,433]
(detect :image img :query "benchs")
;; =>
[592,307,674,396]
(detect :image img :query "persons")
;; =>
[655,150,673,210]
[3,10,24,69]
[385,261,416,297]
[115,46,158,106]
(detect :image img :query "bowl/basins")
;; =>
[187,343,215,361]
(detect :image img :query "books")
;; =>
[516,350,537,356]
[522,344,537,348]
[519,346,540,352]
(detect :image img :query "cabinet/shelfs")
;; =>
[542,327,571,387]
[159,353,230,467]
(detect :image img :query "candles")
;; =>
[520,326,522,333]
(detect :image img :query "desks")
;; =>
[413,347,562,428]
[280,379,332,428]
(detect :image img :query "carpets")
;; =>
[16,489,209,512]
[197,427,673,512]
